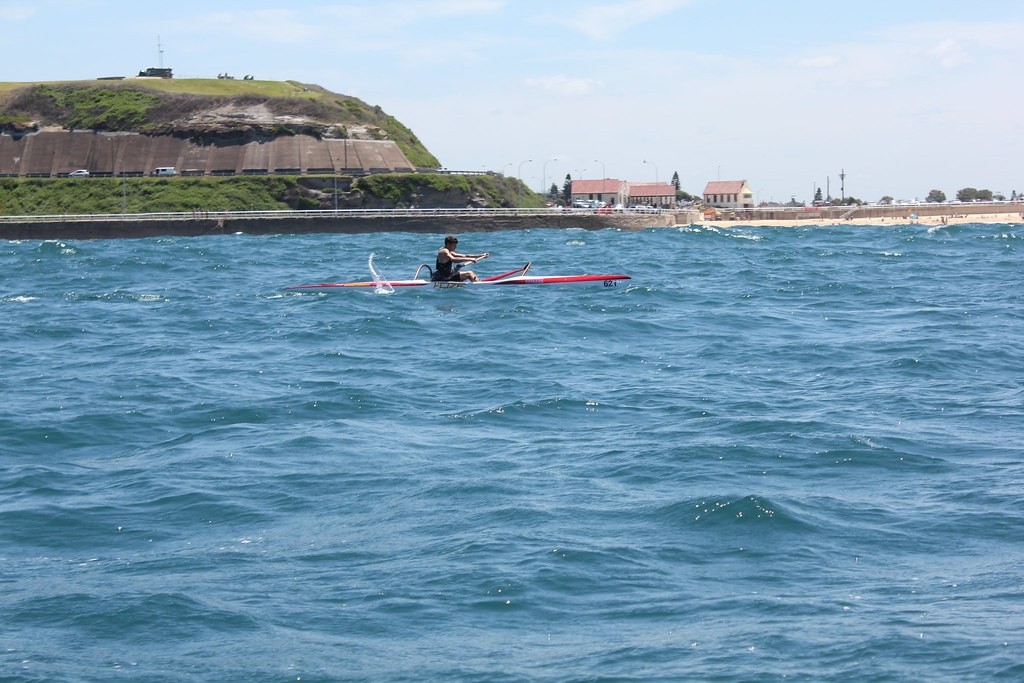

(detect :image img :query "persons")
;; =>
[436,236,487,281]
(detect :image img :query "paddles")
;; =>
[432,256,485,277]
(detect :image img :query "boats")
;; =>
[283,262,631,292]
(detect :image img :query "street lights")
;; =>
[643,161,658,209]
[544,159,557,199]
[519,160,532,207]
[575,169,586,180]
[108,138,114,173]
[594,160,605,201]
[503,163,512,176]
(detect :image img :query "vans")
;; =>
[815,201,835,206]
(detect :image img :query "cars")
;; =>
[153,166,176,176]
[67,170,90,177]
[436,168,450,174]
[613,204,657,214]
[573,200,606,208]
